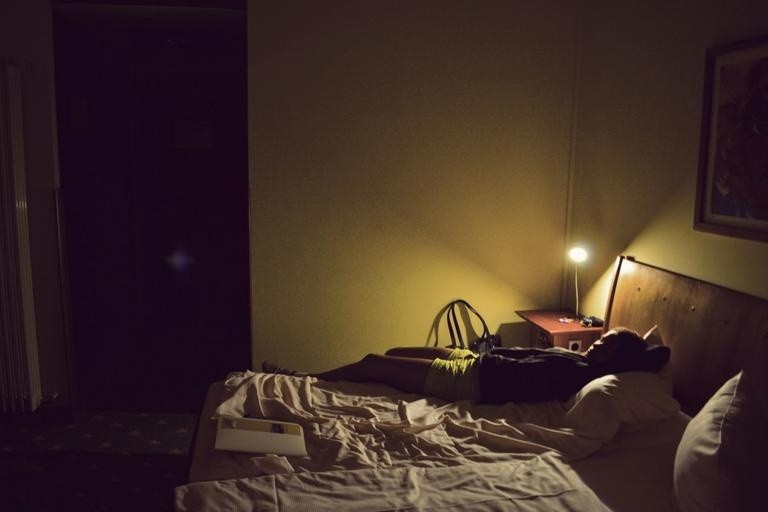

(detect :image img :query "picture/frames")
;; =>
[693,34,768,243]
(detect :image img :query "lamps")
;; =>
[569,246,588,318]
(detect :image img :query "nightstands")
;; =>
[515,308,603,352]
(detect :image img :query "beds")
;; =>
[174,256,768,512]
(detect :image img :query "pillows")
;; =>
[670,369,750,512]
[563,325,682,462]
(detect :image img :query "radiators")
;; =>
[0,60,58,414]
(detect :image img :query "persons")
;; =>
[262,326,671,405]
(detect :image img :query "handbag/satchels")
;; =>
[445,300,502,354]
